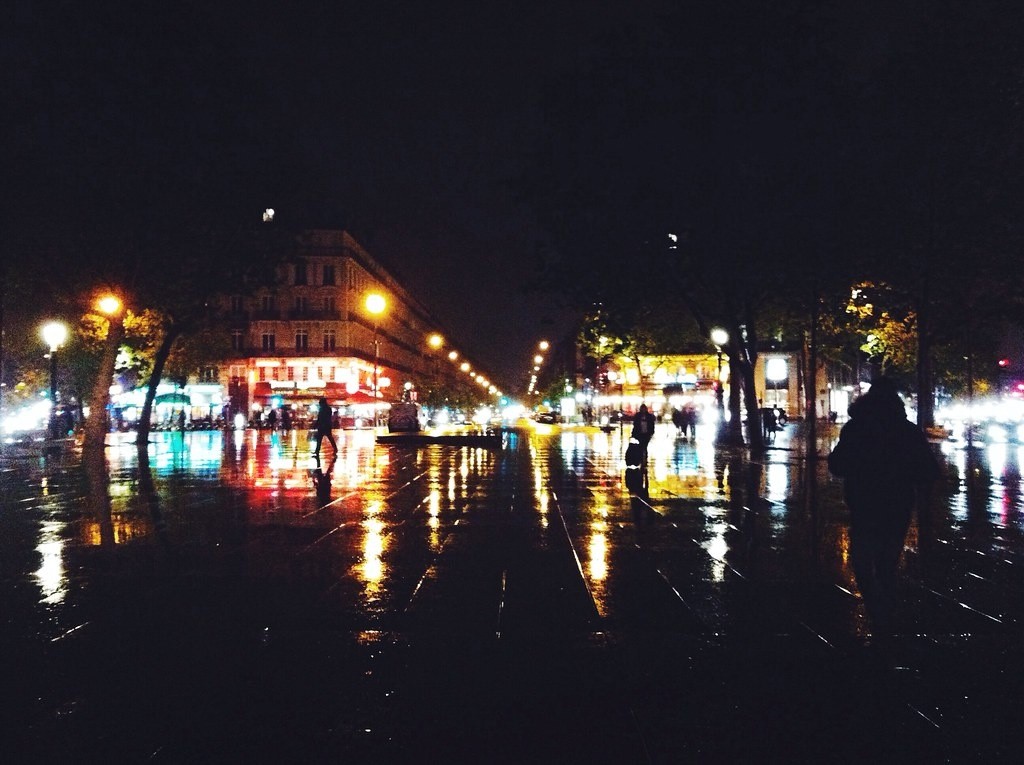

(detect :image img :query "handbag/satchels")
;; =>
[625,437,642,466]
[624,467,643,490]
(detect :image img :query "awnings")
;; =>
[49,401,84,412]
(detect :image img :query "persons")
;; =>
[582,407,593,422]
[201,413,223,423]
[254,407,291,438]
[177,409,186,431]
[672,405,697,440]
[631,405,654,469]
[826,380,937,667]
[46,406,83,438]
[760,400,787,442]
[311,398,338,455]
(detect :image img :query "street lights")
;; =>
[766,359,788,408]
[963,352,976,452]
[826,382,832,422]
[367,293,388,428]
[710,327,730,421]
[41,320,70,421]
